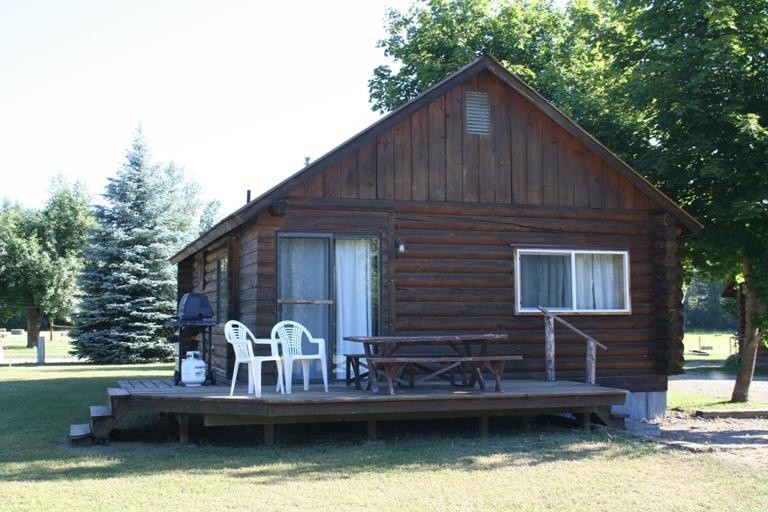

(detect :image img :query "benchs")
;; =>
[343,352,473,388]
[367,355,522,396]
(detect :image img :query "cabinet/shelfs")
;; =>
[164,321,217,386]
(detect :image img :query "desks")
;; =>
[342,334,508,393]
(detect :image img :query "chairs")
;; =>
[270,320,329,394]
[224,320,285,399]
